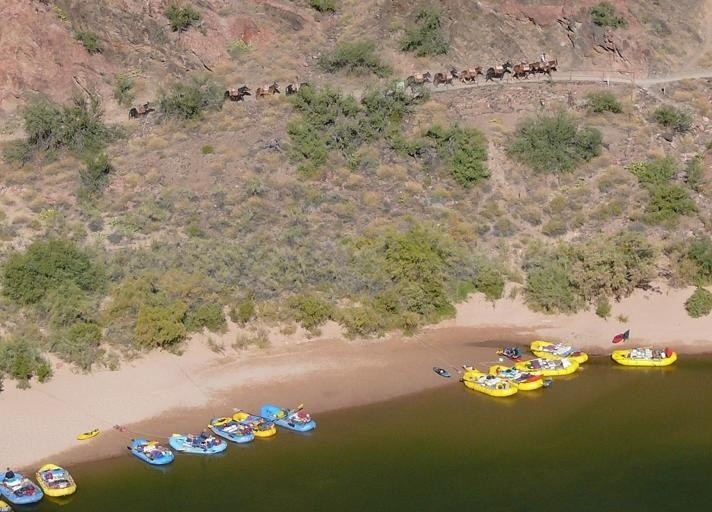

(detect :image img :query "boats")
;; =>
[529,340,588,364]
[169,432,227,455]
[612,346,680,368]
[260,405,316,433]
[516,359,580,375]
[128,437,174,467]
[36,464,77,498]
[233,408,274,439]
[463,368,519,396]
[490,364,546,391]
[209,417,253,442]
[1,469,43,504]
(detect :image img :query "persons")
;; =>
[201,428,208,440]
[540,52,546,63]
[5,466,14,478]
[292,76,300,92]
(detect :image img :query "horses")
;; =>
[256,82,281,98]
[406,57,559,90]
[224,85,252,101]
[129,100,156,118]
[284,82,308,95]
